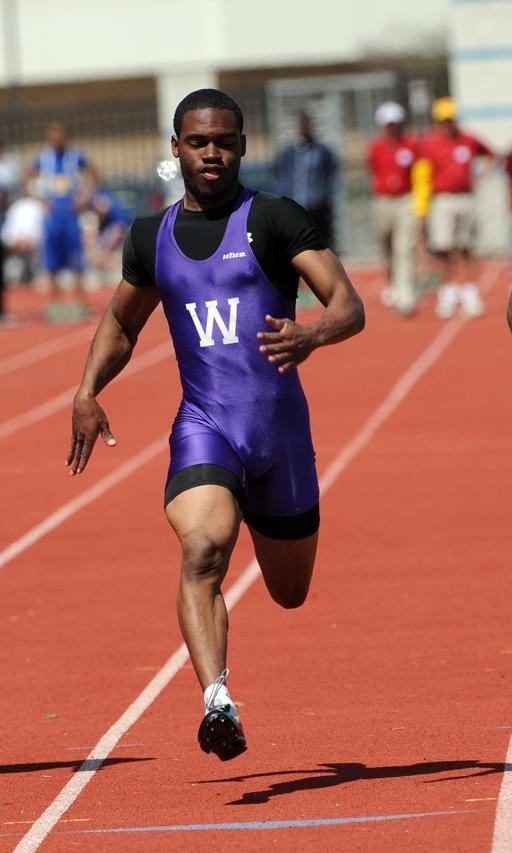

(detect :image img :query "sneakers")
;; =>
[197,668,248,761]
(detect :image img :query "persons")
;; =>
[1,124,130,324]
[66,90,364,759]
[277,112,344,255]
[360,97,504,320]
